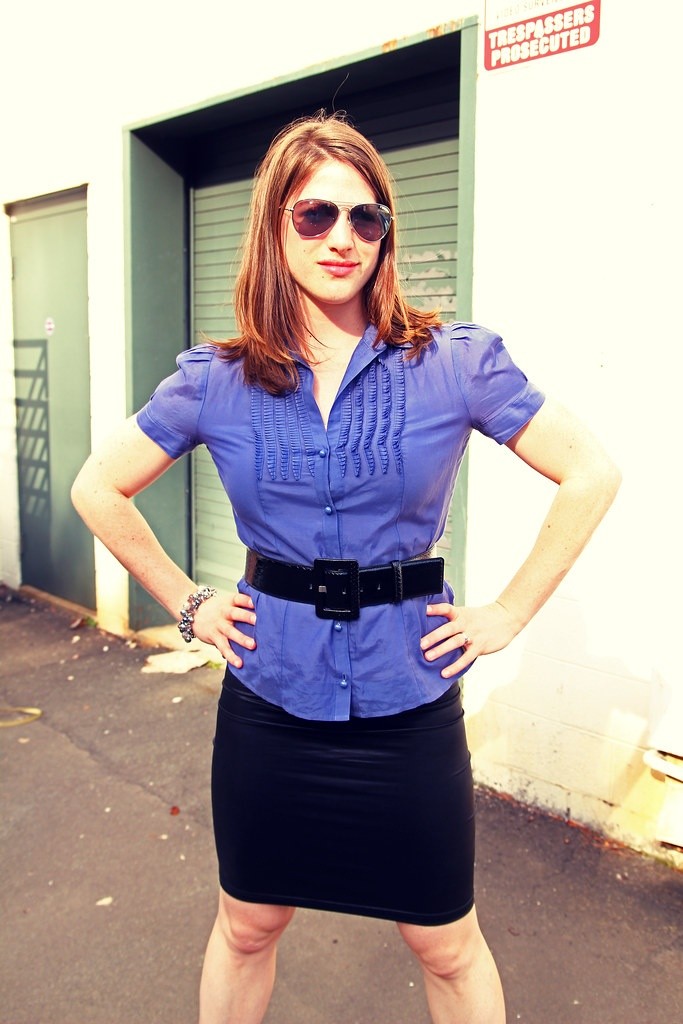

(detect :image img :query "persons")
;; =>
[69,118,623,1024]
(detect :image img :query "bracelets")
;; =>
[177,584,217,644]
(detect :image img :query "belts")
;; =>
[245,547,444,620]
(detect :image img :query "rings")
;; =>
[462,633,470,645]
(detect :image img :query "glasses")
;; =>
[284,199,395,242]
